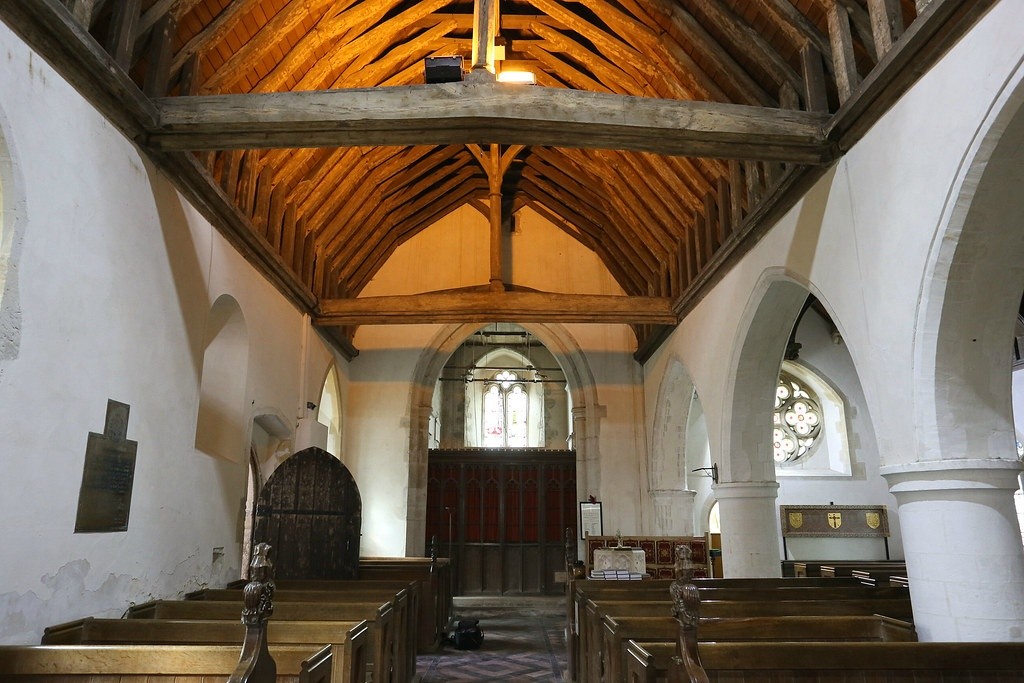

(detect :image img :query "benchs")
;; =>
[0,557,450,683]
[566,559,1024,683]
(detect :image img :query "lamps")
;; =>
[425,55,464,84]
[496,70,536,85]
